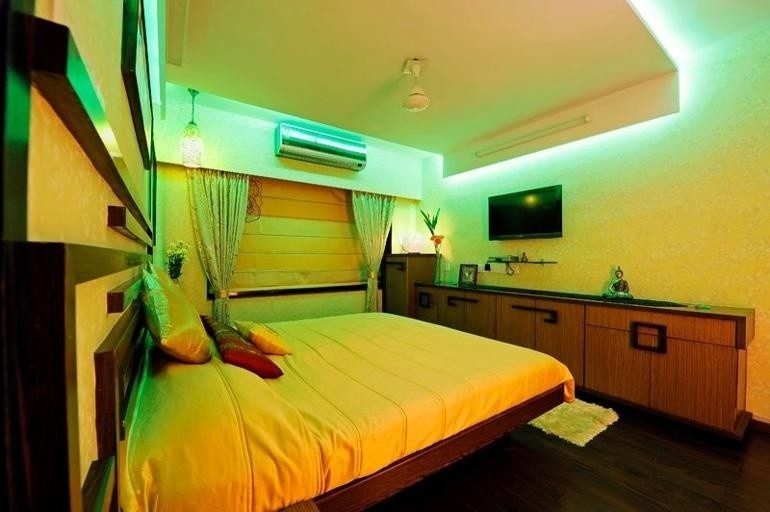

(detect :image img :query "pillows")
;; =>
[142,261,293,379]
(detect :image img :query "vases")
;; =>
[434,248,442,283]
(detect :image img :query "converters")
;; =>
[485,264,490,270]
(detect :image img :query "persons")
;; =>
[603,265,633,300]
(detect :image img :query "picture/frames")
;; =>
[458,264,477,286]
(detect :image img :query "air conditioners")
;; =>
[275,123,367,171]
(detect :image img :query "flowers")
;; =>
[167,240,190,279]
[420,208,445,248]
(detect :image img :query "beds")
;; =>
[95,312,576,512]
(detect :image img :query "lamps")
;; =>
[183,88,202,168]
[472,114,592,158]
[401,58,429,112]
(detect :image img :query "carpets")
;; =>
[527,399,619,447]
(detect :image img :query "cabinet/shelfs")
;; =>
[438,282,494,339]
[414,286,438,324]
[383,257,437,318]
[584,303,737,438]
[495,296,584,398]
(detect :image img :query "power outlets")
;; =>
[478,263,519,274]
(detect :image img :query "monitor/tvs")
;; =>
[488,184,562,240]
